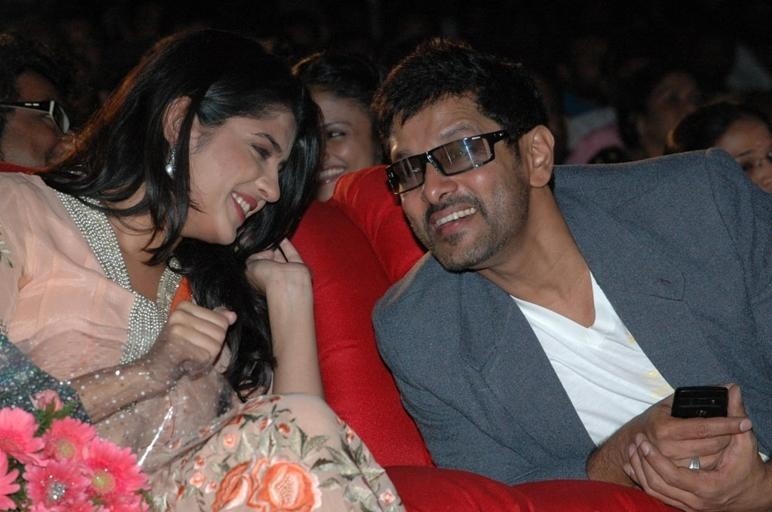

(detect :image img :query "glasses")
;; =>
[4,98,71,136]
[384,130,510,195]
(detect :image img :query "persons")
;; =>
[287,48,388,205]
[530,26,770,194]
[1,32,81,168]
[1,29,408,510]
[369,42,771,510]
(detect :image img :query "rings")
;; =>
[688,454,701,471]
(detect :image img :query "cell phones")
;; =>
[671,386,731,421]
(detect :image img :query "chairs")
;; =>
[289,163,681,512]
